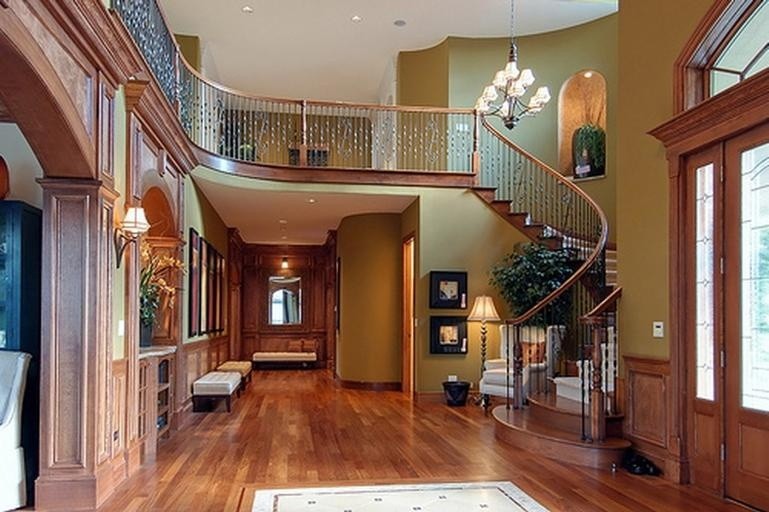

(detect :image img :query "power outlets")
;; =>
[652,321,663,338]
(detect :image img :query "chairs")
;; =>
[0,351,32,512]
[481,324,564,412]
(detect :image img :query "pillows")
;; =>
[302,339,317,353]
[286,340,302,352]
[519,341,545,363]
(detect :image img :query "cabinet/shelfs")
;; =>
[131,344,179,445]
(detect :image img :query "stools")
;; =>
[192,372,243,412]
[216,361,253,389]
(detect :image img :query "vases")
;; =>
[139,315,153,348]
[571,124,606,178]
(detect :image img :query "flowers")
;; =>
[138,240,189,324]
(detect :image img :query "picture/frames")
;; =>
[428,270,468,308]
[429,315,469,355]
[188,227,234,337]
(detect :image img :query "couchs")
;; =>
[252,334,319,370]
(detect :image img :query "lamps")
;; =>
[473,0,552,130]
[468,295,501,408]
[281,260,289,269]
[121,206,149,236]
